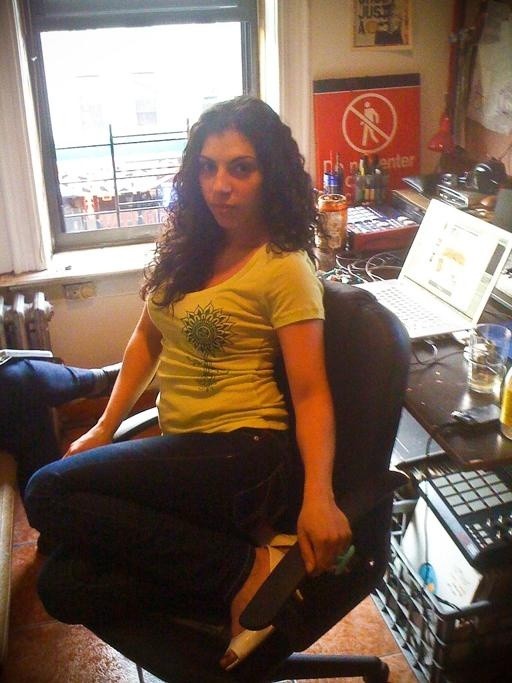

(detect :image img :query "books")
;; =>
[2,349,54,365]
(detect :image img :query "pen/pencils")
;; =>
[323,151,339,174]
[355,155,382,206]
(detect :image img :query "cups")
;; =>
[467,323,512,394]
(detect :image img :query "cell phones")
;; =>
[451,404,501,435]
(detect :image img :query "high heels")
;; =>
[220,545,306,673]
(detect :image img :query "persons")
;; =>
[2,357,126,502]
[19,94,354,670]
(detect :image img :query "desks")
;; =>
[320,249,512,471]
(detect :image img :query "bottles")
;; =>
[500,363,512,443]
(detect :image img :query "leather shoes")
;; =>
[87,362,122,400]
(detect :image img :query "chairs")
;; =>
[38,283,412,683]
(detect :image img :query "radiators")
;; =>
[0,290,55,354]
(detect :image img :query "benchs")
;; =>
[1,452,17,678]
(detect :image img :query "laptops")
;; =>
[350,197,512,348]
